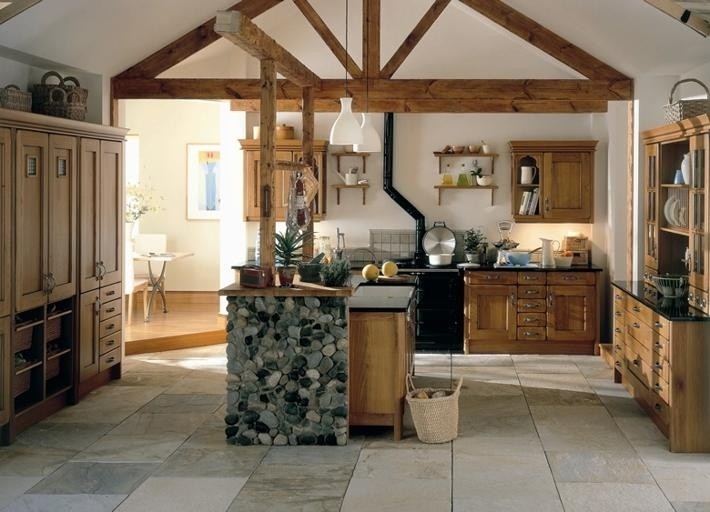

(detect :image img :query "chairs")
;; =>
[137,232,170,302]
[124,231,147,325]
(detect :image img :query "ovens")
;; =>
[413,271,465,352]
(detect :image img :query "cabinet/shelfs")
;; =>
[517,271,547,354]
[330,152,372,205]
[547,269,601,356]
[462,270,518,354]
[609,273,710,453]
[505,139,600,224]
[238,137,329,224]
[433,150,500,208]
[343,275,420,441]
[639,113,710,315]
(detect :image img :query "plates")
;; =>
[457,263,481,266]
[663,196,687,228]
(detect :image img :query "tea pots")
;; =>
[538,237,560,265]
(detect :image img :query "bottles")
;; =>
[442,163,454,185]
[318,236,343,260]
[471,160,479,175]
[456,164,468,186]
[680,153,691,185]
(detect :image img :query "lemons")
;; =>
[565,251,571,257]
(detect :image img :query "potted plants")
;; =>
[462,227,489,265]
[268,225,322,287]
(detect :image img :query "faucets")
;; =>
[348,246,379,267]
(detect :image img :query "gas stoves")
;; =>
[388,259,460,273]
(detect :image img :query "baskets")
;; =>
[1,84,35,113]
[405,374,463,444]
[41,87,88,122]
[32,71,89,117]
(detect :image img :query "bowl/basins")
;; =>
[298,262,322,282]
[492,241,520,250]
[554,256,573,266]
[477,175,493,186]
[468,145,482,153]
[452,146,465,154]
[427,253,455,265]
[654,297,690,317]
[508,253,532,266]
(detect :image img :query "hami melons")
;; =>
[381,261,398,277]
[362,264,379,281]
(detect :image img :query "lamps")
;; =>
[327,0,366,146]
[350,1,383,153]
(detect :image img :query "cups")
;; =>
[253,126,260,141]
[483,144,492,154]
[674,169,685,184]
[521,165,536,184]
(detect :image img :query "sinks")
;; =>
[350,266,382,271]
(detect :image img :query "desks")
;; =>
[134,250,199,316]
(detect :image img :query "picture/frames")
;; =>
[185,143,221,223]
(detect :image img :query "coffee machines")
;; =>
[496,221,513,265]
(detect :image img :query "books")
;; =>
[527,188,539,217]
[518,191,532,216]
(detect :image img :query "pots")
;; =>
[276,123,294,139]
